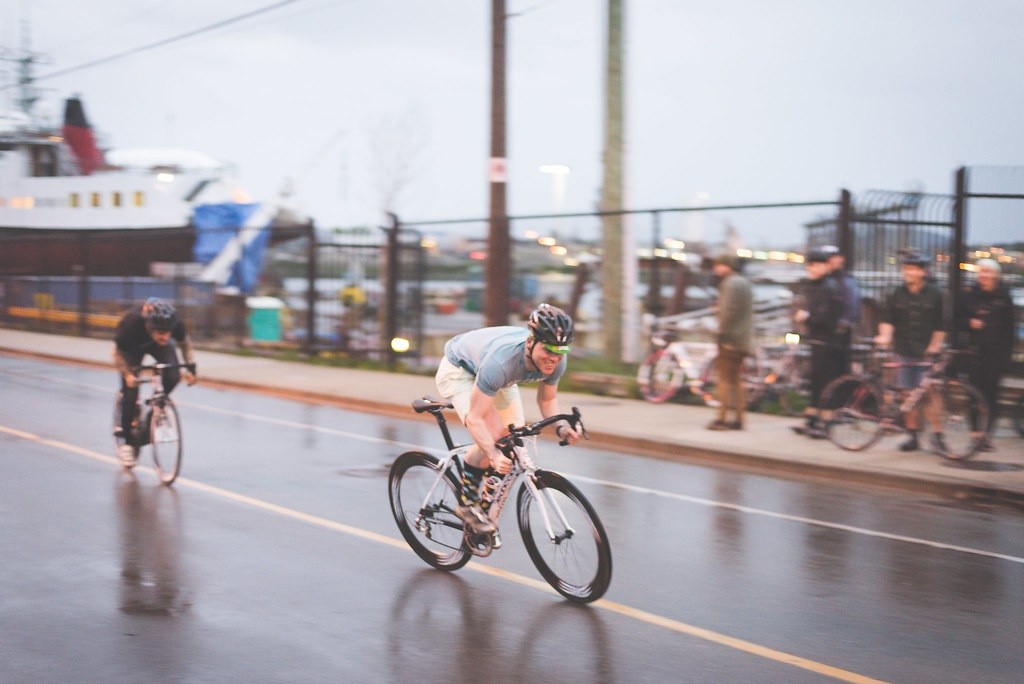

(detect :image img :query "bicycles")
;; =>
[111,363,198,486]
[820,340,992,460]
[388,396,612,602]
[637,316,767,408]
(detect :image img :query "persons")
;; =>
[793,248,846,438]
[794,245,859,425]
[953,259,1015,452]
[113,296,197,470]
[700,253,754,431]
[432,302,583,549]
[875,251,950,453]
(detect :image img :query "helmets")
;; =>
[141,297,180,331]
[529,303,575,354]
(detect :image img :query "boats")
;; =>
[0,0,201,275]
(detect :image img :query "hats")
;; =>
[713,255,738,272]
[898,248,931,267]
[821,245,842,257]
[804,248,827,262]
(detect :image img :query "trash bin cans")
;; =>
[244,297,283,341]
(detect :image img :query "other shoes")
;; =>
[119,443,136,467]
[491,531,502,550]
[931,432,947,452]
[808,422,829,439]
[899,438,919,451]
[456,504,495,533]
[728,421,742,430]
[708,420,727,430]
[972,436,994,452]
[792,419,815,434]
